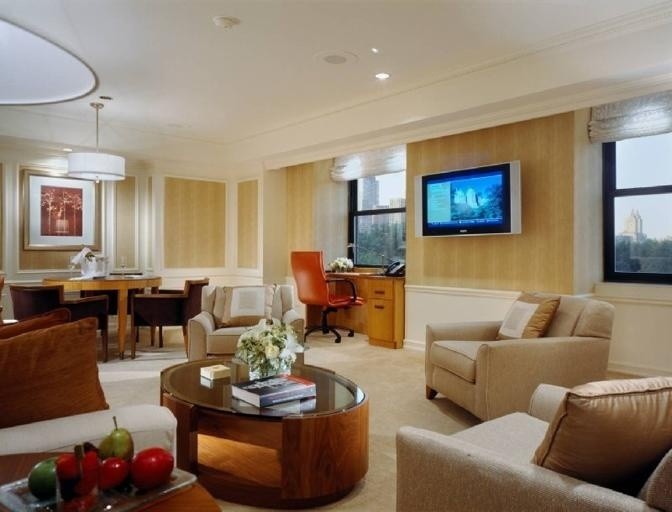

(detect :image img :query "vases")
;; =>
[249,354,291,381]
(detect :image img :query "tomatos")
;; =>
[58,450,98,500]
[101,456,126,488]
[129,448,175,490]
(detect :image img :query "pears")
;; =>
[98,428,134,461]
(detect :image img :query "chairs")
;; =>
[130,276,210,360]
[394,380,671,512]
[9,285,108,363]
[425,292,615,424]
[80,273,143,345]
[289,251,368,344]
[187,285,304,364]
[0,402,178,470]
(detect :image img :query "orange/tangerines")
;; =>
[28,459,57,500]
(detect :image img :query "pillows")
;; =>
[1,315,110,430]
[531,375,672,474]
[496,289,561,344]
[2,306,76,344]
[221,285,278,326]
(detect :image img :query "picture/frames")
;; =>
[22,169,101,252]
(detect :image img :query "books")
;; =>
[231,372,318,408]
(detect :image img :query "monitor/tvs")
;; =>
[415,160,522,237]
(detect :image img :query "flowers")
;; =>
[328,255,353,272]
[237,314,311,380]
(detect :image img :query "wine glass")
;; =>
[66,255,129,280]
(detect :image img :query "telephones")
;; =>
[385,261,405,276]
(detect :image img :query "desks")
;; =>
[305,271,405,349]
[40,274,163,360]
[0,452,226,512]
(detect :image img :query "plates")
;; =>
[0,467,197,512]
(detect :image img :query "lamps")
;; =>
[67,100,128,184]
